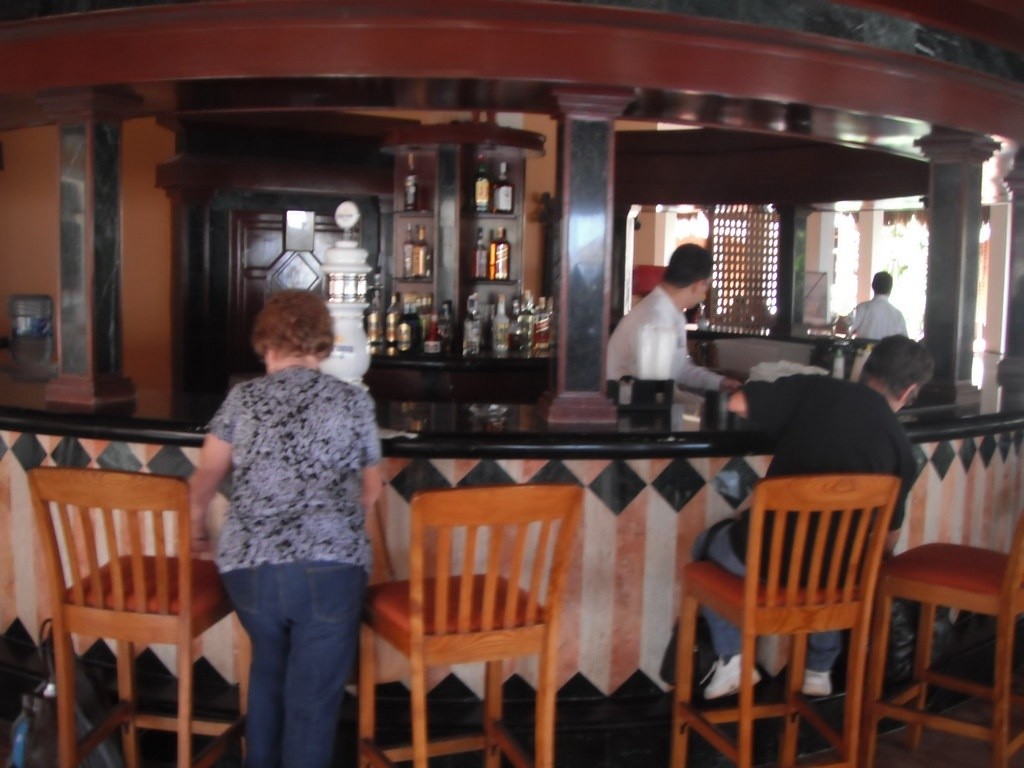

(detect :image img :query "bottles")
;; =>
[411,225,431,279]
[473,155,491,211]
[492,294,510,357]
[366,291,383,354]
[437,302,454,351]
[511,299,520,357]
[462,295,480,356]
[492,162,514,213]
[387,290,437,357]
[490,227,510,281]
[535,298,553,349]
[471,228,487,278]
[403,223,415,278]
[516,290,538,358]
[9,295,51,368]
[403,153,420,211]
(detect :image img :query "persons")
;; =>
[605,242,745,404]
[184,289,382,768]
[686,333,934,700]
[835,269,908,343]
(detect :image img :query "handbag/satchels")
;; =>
[5,617,127,768]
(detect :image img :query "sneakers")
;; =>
[704,654,761,700]
[802,669,832,695]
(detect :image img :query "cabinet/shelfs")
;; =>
[378,122,547,351]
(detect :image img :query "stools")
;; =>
[856,505,1024,768]
[24,463,253,768]
[357,484,584,768]
[666,472,902,768]
